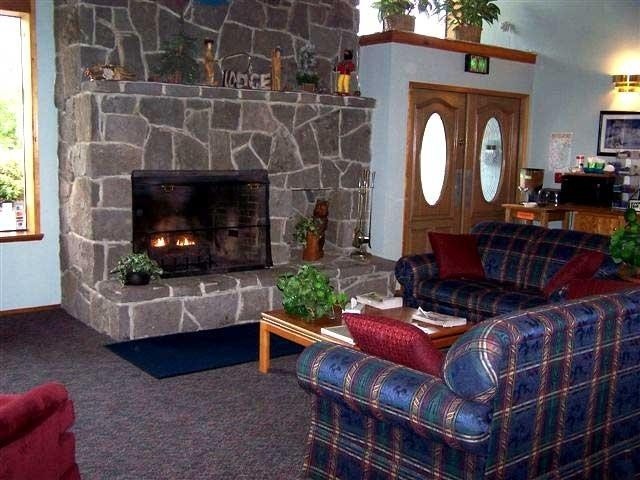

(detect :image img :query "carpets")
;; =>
[105,320,301,382]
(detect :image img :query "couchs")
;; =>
[394,221,622,325]
[291,289,640,479]
[0,380,85,480]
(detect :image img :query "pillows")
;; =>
[565,278,640,302]
[543,248,604,295]
[341,309,442,375]
[429,227,485,284]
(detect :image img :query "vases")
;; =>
[301,81,314,92]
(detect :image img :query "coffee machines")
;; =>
[518,165,544,206]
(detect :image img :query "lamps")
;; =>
[612,76,639,94]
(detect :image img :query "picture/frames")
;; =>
[628,199,640,212]
[597,110,640,159]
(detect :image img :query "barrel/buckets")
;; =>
[520,168,543,193]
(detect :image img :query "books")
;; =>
[356,290,403,310]
[411,311,467,327]
[321,325,355,346]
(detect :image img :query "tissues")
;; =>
[341,297,365,314]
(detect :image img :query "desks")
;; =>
[500,201,640,235]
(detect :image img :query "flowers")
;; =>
[297,43,322,81]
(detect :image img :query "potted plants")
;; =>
[278,264,343,323]
[109,250,167,288]
[603,208,640,286]
[432,0,500,42]
[292,200,330,262]
[370,0,415,32]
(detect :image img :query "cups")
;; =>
[573,153,618,173]
